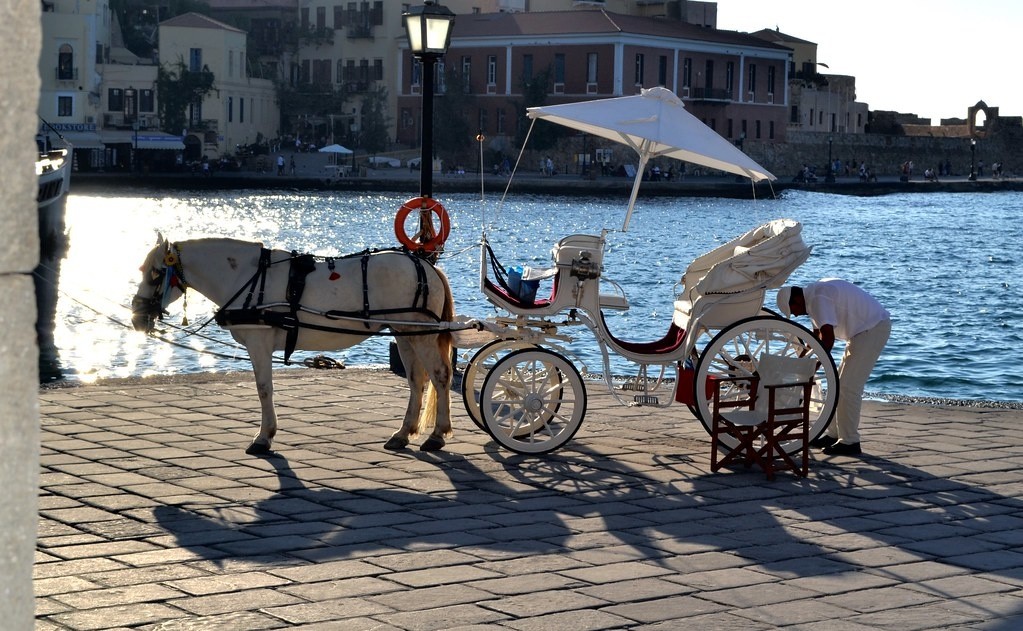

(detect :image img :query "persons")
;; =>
[187,131,729,181]
[777,278,892,456]
[791,156,1003,185]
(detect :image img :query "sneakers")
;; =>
[822,440,861,455]
[811,435,839,447]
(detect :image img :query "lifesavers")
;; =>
[394,197,450,252]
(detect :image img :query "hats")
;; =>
[776,286,791,319]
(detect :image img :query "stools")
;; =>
[673,285,767,331]
[599,294,630,310]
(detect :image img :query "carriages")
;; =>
[131,218,842,481]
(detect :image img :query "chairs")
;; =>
[337,166,344,177]
[710,351,817,484]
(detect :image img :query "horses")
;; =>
[131,232,456,454]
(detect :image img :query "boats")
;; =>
[36,115,75,249]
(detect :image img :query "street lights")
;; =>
[328,113,358,167]
[739,131,747,153]
[386,1,459,379]
[824,135,836,185]
[131,123,140,174]
[967,138,979,179]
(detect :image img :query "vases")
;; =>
[664,172,668,177]
